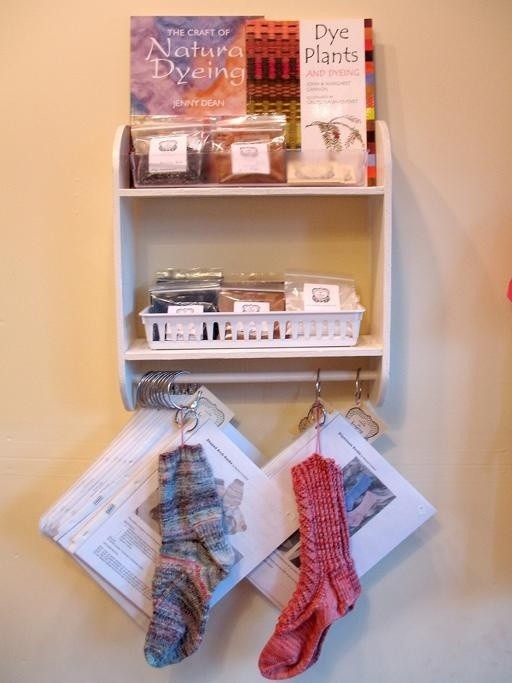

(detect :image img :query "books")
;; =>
[245,410,438,613]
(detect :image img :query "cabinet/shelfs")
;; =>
[112,124,393,412]
